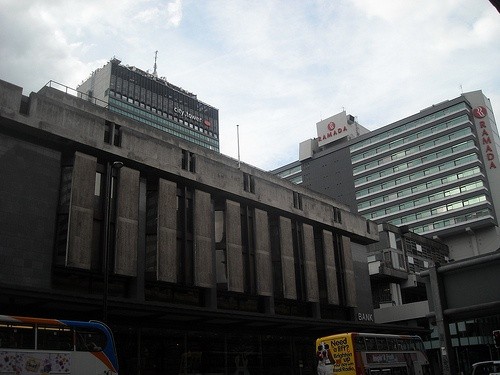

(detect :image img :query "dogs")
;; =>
[316,341,336,375]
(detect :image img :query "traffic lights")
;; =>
[492,330,500,349]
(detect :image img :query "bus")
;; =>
[0,315,119,375]
[316,332,432,375]
[469,360,500,375]
